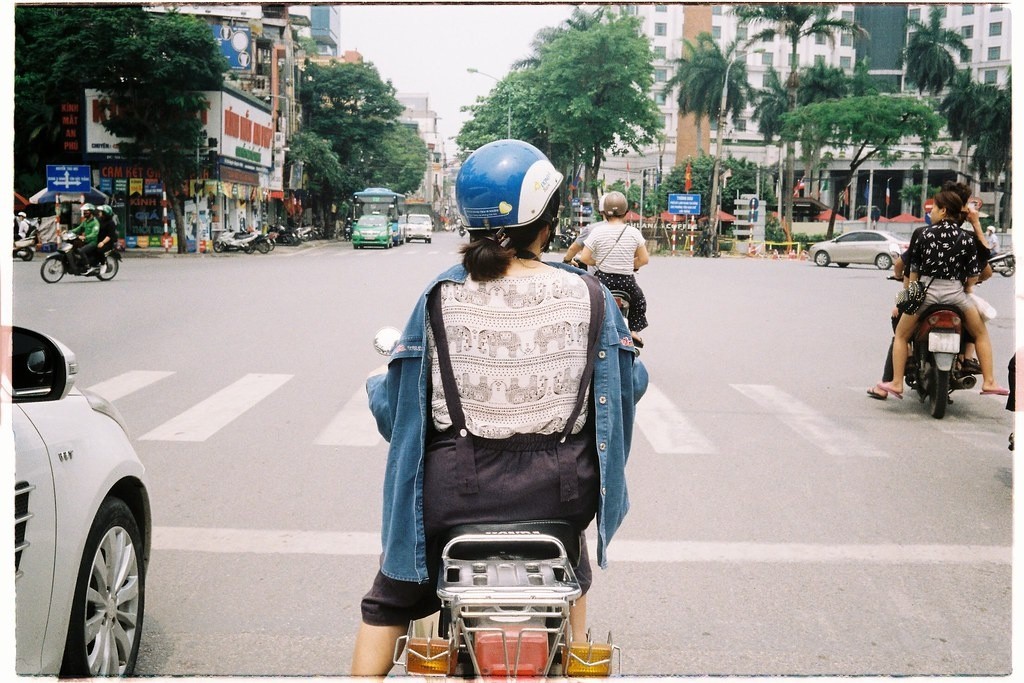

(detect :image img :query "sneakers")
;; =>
[962,357,982,374]
[904,357,920,376]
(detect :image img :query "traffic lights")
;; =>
[643,170,649,200]
[633,202,639,211]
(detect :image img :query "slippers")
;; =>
[632,337,643,348]
[877,382,903,399]
[980,387,1009,396]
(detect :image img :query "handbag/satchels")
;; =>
[896,281,925,315]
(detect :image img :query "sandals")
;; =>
[866,387,888,399]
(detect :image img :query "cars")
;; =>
[352,215,394,250]
[807,230,912,270]
[11,326,153,678]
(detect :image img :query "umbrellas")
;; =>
[29,186,109,204]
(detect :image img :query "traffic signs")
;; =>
[44,164,92,194]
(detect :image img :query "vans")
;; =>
[407,214,434,243]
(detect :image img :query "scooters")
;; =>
[991,251,1017,277]
[13,235,39,262]
[343,225,351,242]
[458,230,465,237]
[373,325,623,675]
[569,258,631,326]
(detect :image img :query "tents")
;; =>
[627,211,736,222]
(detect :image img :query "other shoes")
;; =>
[100,264,108,275]
[81,267,100,276]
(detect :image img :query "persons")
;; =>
[62,203,115,273]
[986,225,999,254]
[350,139,650,683]
[562,191,649,348]
[867,181,1010,400]
[14,212,30,240]
[312,214,321,228]
[345,218,352,228]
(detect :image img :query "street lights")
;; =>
[702,49,767,259]
[466,67,511,140]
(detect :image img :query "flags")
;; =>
[571,171,580,190]
[625,163,631,191]
[685,163,691,190]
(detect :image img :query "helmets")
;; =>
[97,205,113,215]
[80,203,95,210]
[599,191,628,215]
[455,140,563,231]
[18,211,27,217]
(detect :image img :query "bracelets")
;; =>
[564,257,572,262]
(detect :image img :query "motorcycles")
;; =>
[213,217,321,255]
[39,233,123,284]
[887,274,980,421]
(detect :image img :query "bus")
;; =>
[353,187,407,246]
